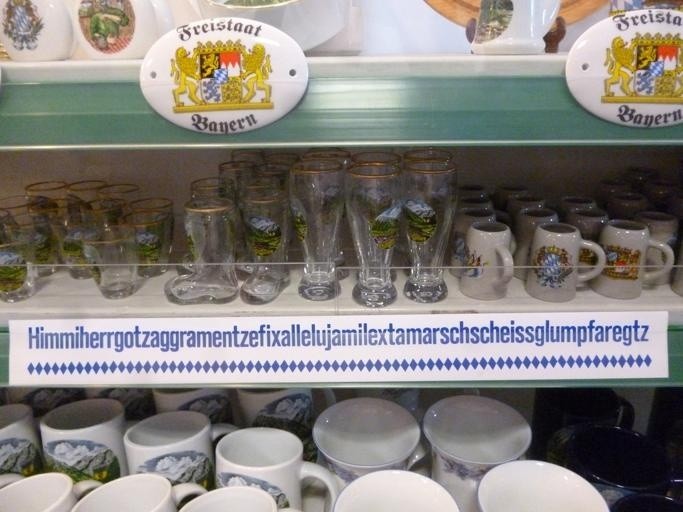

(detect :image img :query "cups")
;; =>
[0,384,682,512]
[473,0,560,54]
[0,177,172,299]
[450,169,683,302]
[0,0,159,62]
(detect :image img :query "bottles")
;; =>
[287,147,351,302]
[218,150,290,310]
[163,178,240,306]
[402,146,457,304]
[344,151,401,310]
